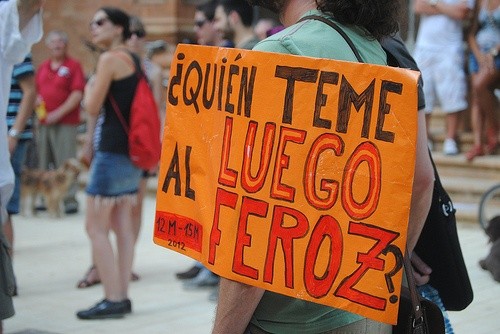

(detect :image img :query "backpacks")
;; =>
[95,50,162,170]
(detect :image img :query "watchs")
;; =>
[8,128,18,140]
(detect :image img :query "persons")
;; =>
[0,0,48,334]
[35,28,86,213]
[74,0,500,334]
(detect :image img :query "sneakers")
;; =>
[183,270,219,289]
[427,138,435,152]
[175,266,201,280]
[443,139,459,155]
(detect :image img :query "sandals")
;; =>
[78,266,101,288]
[130,274,140,282]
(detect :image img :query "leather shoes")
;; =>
[76,299,132,319]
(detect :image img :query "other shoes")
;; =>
[466,144,485,162]
[34,194,46,209]
[488,136,500,153]
[65,196,79,212]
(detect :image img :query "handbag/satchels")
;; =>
[414,180,473,312]
[392,284,446,334]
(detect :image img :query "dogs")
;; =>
[18,158,80,219]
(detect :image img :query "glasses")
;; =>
[90,20,105,26]
[195,19,208,27]
